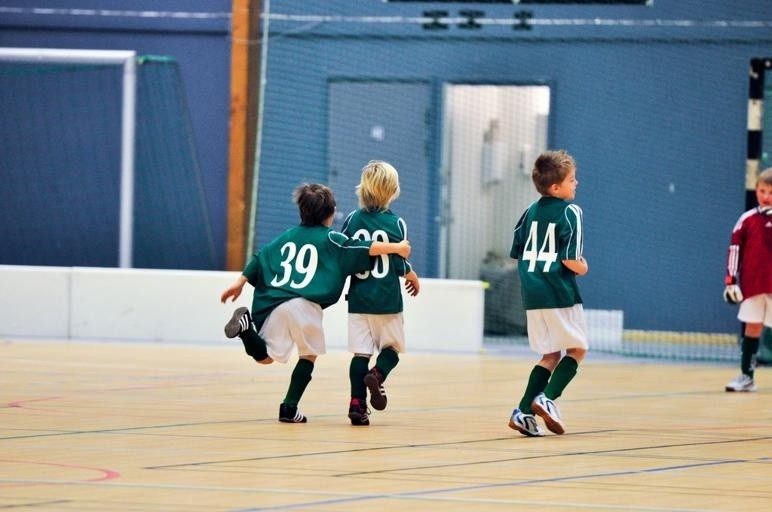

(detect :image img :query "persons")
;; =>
[219,182,412,424]
[508,148,590,438]
[721,168,772,391]
[341,159,420,426]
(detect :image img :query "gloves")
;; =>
[719,276,746,306]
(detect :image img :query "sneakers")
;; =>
[725,373,761,394]
[508,408,547,438]
[531,391,566,436]
[225,306,252,338]
[364,368,389,412]
[346,398,371,426]
[278,403,308,425]
